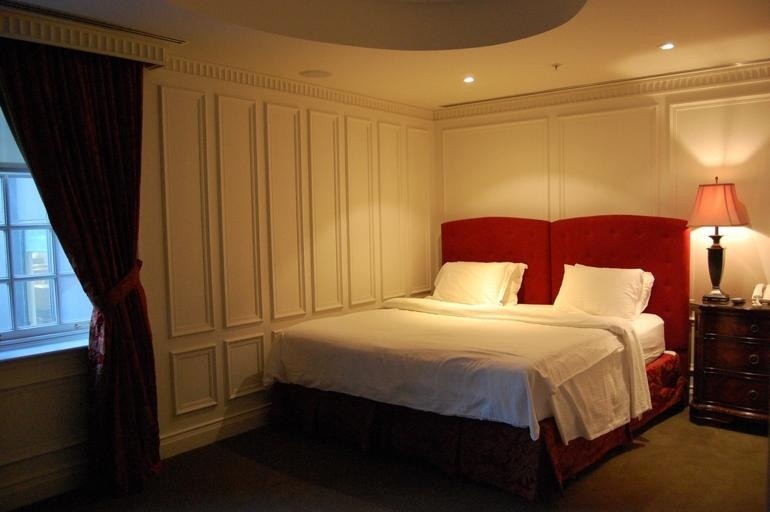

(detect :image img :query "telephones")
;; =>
[752,284,770,302]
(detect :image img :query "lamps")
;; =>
[689,181,750,303]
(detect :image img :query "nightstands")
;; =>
[689,297,770,439]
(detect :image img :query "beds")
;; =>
[265,215,689,512]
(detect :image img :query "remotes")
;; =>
[731,297,745,304]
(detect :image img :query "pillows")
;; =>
[431,261,528,304]
[553,262,656,319]
